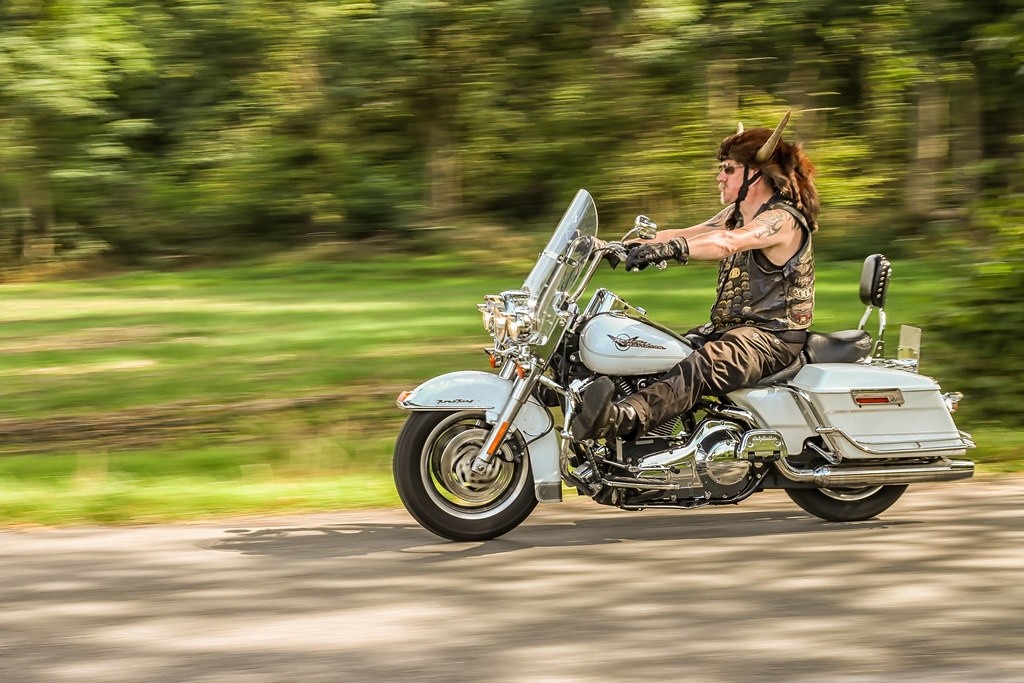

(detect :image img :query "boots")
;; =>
[571,376,637,440]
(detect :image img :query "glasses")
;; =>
[719,165,744,174]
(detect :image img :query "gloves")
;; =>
[625,236,690,271]
[574,235,620,270]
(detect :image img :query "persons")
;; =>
[572,128,820,442]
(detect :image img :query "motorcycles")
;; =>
[392,186,978,542]
